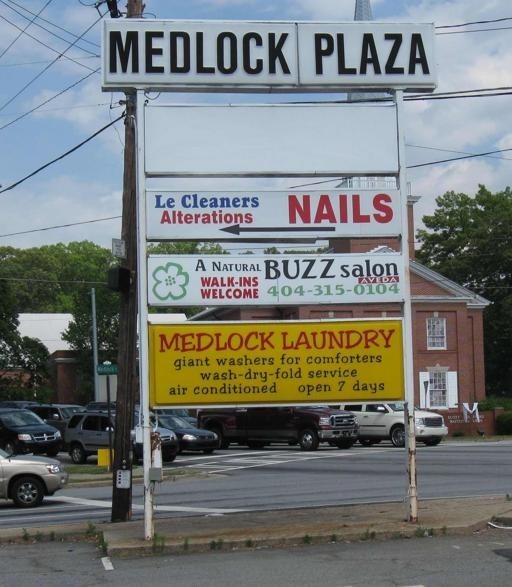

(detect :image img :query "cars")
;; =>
[199,403,360,451]
[0,396,236,465]
[0,446,70,507]
[335,401,450,447]
